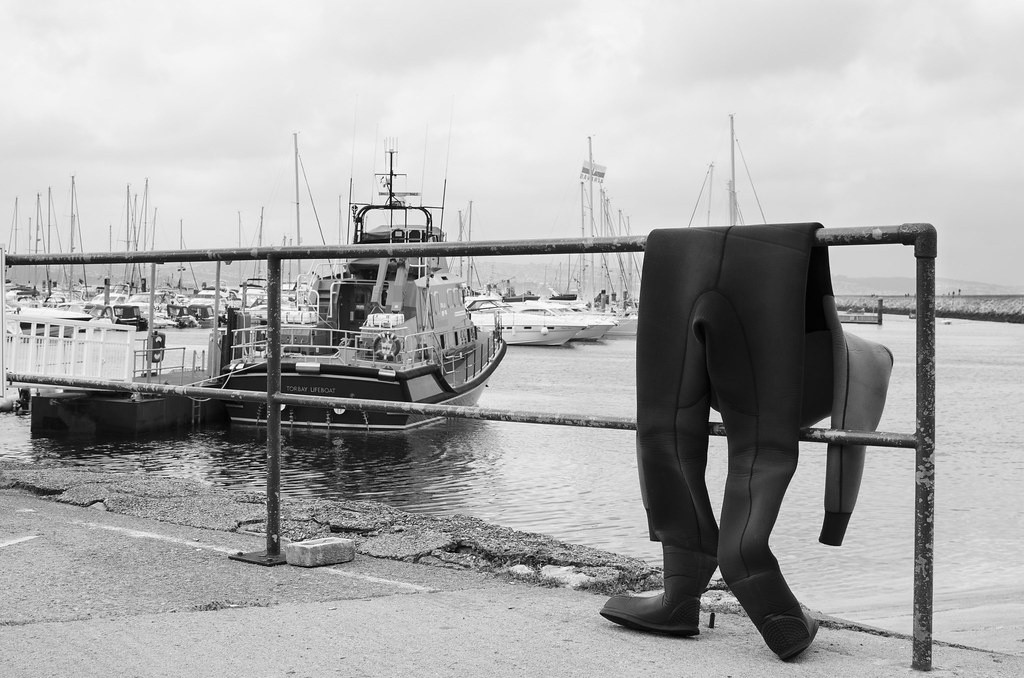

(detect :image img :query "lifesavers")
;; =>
[373,331,401,360]
[146,332,166,363]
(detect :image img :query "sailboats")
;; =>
[448,134,639,346]
[0,168,344,434]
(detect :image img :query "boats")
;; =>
[223,130,509,444]
